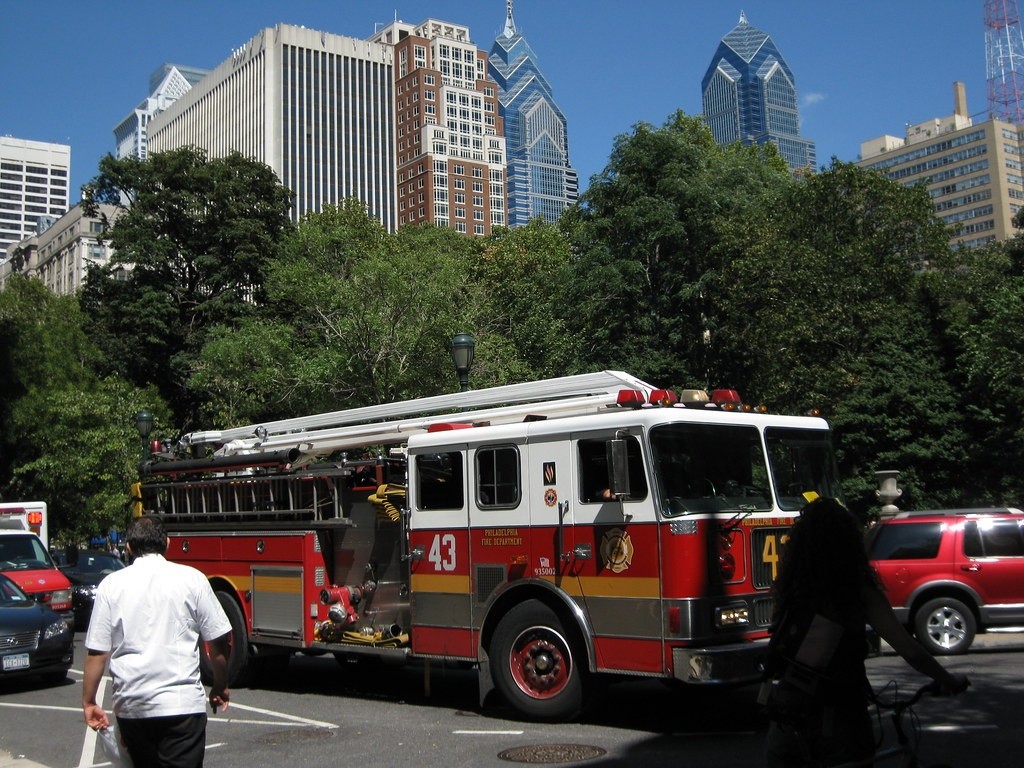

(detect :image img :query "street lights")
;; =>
[444,333,476,391]
[133,409,153,462]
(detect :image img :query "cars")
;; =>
[47,548,126,633]
[0,571,74,688]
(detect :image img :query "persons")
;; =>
[83,516,233,768]
[759,497,972,768]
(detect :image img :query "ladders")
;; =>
[140,467,352,534]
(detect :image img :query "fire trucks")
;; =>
[119,369,840,723]
[0,501,77,642]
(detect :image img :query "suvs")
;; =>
[864,507,1023,656]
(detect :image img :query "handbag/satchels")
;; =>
[765,591,866,703]
[97,724,134,768]
